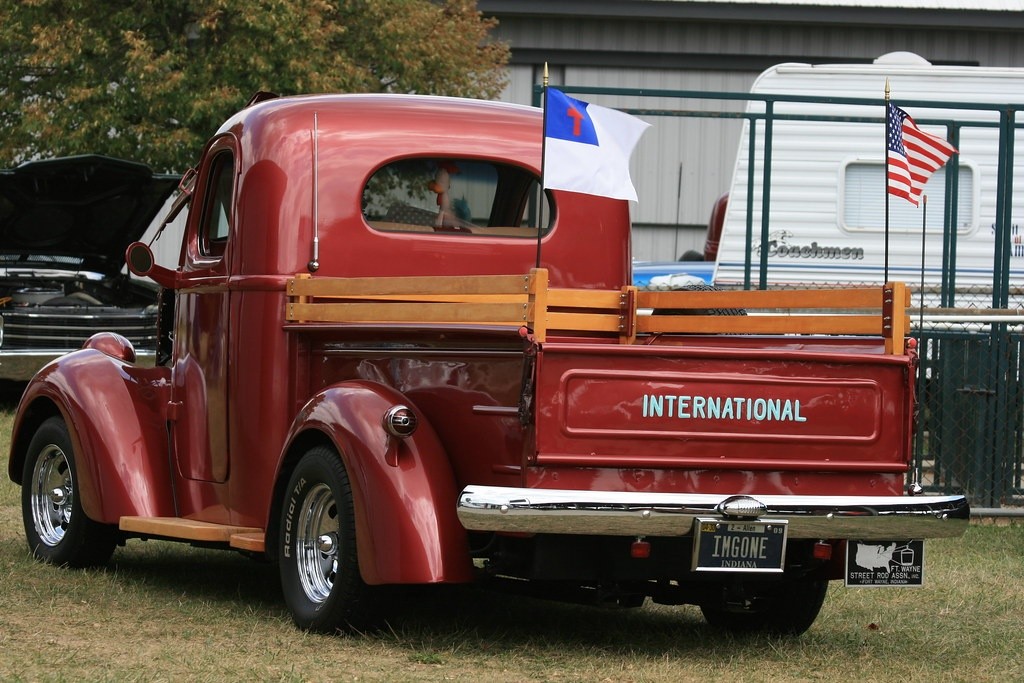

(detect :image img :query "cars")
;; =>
[1,153,193,381]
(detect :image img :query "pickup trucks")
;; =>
[8,84,973,639]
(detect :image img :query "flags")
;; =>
[543,86,653,203]
[886,102,959,208]
